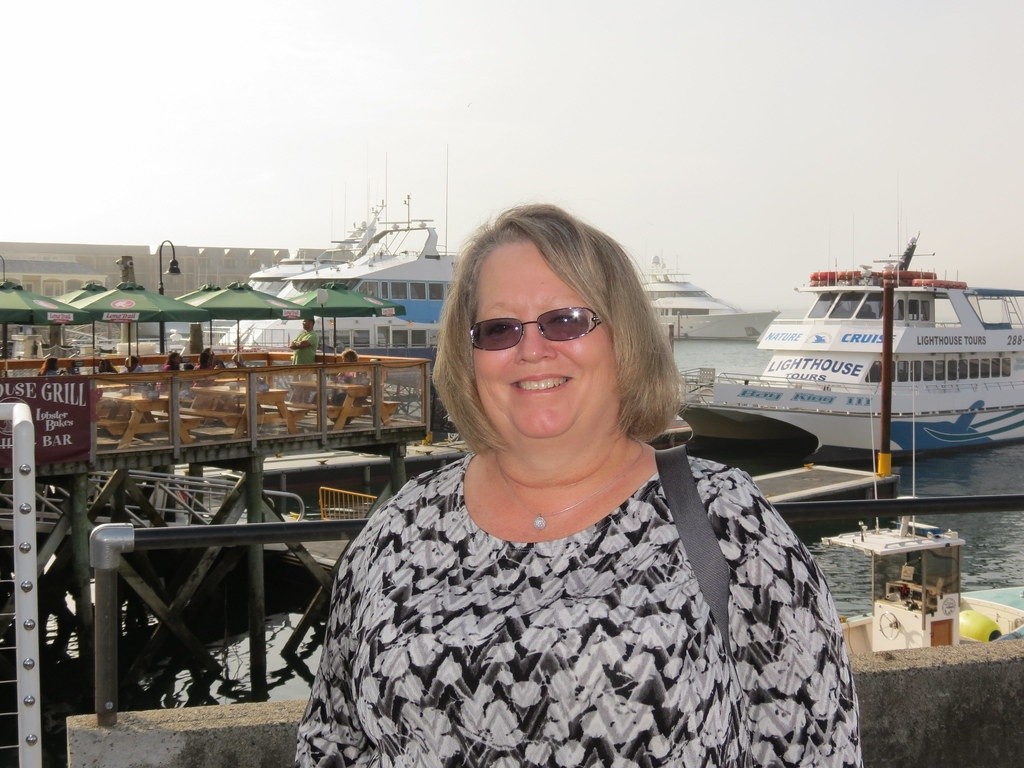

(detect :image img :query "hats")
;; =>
[232,352,243,363]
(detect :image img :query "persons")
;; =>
[99,360,117,374]
[290,319,319,401]
[121,356,159,397]
[37,357,58,376]
[297,205,863,768]
[157,349,248,396]
[332,349,368,406]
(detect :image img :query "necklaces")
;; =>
[497,440,642,530]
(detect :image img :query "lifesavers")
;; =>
[809,270,967,288]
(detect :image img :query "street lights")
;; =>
[156,240,180,353]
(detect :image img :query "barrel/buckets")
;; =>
[959,610,1002,642]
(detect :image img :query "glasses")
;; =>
[471,305,600,352]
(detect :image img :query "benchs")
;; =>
[93,375,400,450]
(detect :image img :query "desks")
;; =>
[290,380,392,429]
[100,390,184,449]
[189,385,299,440]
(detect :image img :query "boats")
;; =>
[639,253,782,343]
[677,251,1024,466]
[116,141,465,379]
[821,360,1024,655]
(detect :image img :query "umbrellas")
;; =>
[0,281,89,360]
[58,281,406,364]
